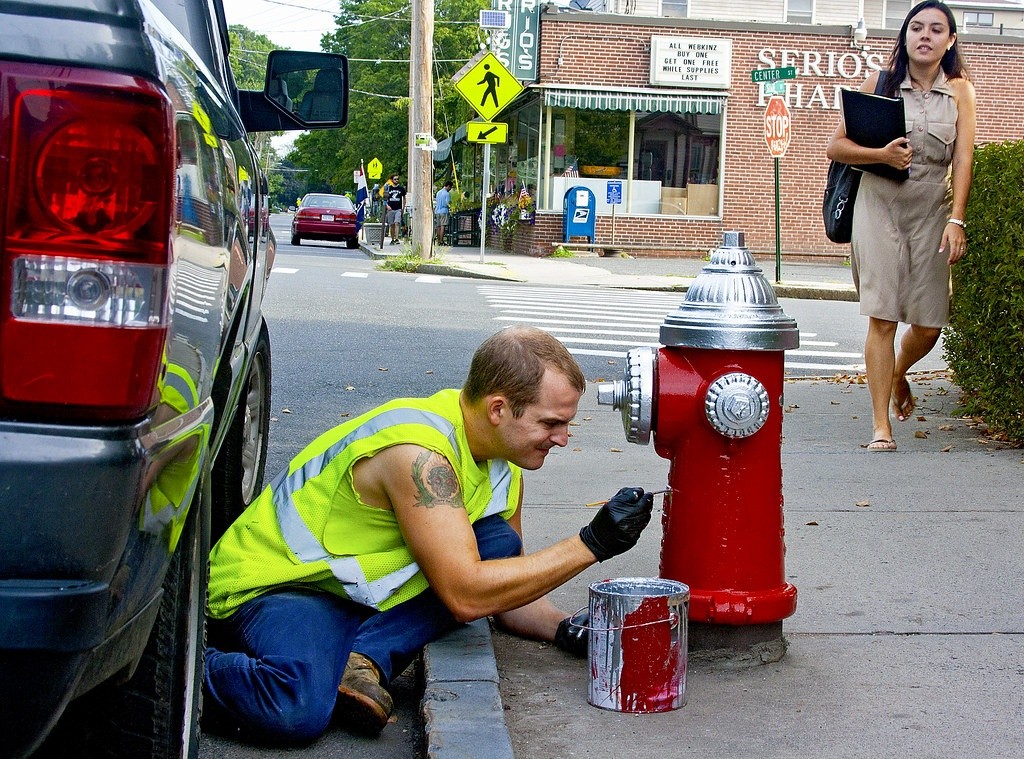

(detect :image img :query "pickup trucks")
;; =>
[0,0,352,759]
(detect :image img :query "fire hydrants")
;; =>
[592,230,800,625]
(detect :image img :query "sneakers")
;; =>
[341,650,393,726]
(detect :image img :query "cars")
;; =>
[287,192,361,249]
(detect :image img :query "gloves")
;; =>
[580,486,653,562]
[555,614,590,655]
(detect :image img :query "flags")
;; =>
[565,159,579,179]
[519,181,529,208]
[497,184,506,201]
[356,163,368,234]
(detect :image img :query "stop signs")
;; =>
[763,96,791,159]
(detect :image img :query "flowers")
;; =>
[487,193,535,228]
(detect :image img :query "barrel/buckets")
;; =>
[588,577,690,713]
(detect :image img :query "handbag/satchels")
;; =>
[821,151,859,246]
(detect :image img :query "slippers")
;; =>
[868,440,896,452]
[892,386,915,423]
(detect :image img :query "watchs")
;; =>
[946,218,967,230]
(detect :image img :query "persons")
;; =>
[434,181,453,245]
[138,336,215,552]
[197,323,657,744]
[384,174,407,244]
[826,0,982,451]
